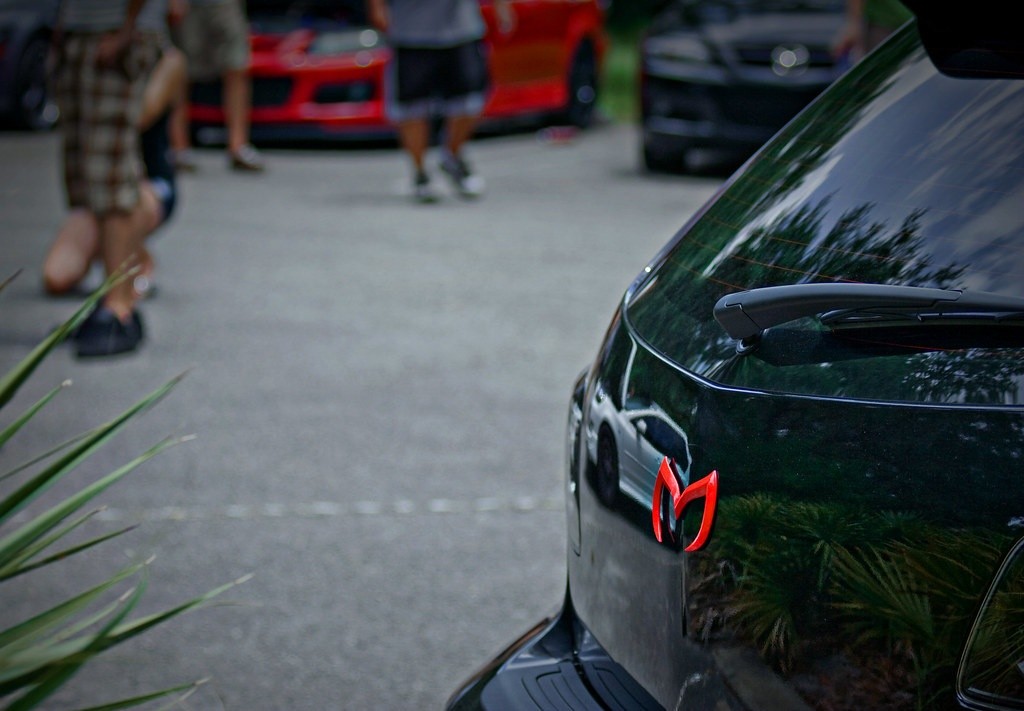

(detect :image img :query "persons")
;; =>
[369,0,491,203]
[41,1,266,359]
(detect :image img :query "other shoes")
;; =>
[416,175,438,202]
[76,310,142,356]
[234,145,264,170]
[438,149,486,197]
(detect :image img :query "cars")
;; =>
[635,2,875,171]
[185,0,604,146]
[440,0,1024,711]
